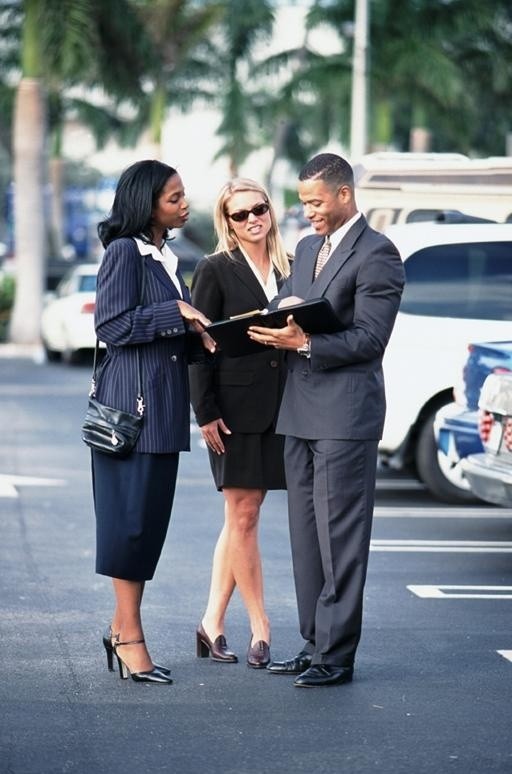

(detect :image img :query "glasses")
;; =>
[226,200,270,222]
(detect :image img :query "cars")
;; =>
[38,265,109,368]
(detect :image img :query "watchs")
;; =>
[297,332,311,357]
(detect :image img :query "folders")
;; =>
[204,297,346,359]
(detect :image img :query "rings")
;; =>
[264,341,267,345]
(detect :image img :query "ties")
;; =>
[314,239,331,281]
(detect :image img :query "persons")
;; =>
[186,176,298,668]
[248,152,408,685]
[87,157,221,683]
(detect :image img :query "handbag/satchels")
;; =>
[81,396,145,456]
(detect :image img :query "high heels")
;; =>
[104,622,353,687]
